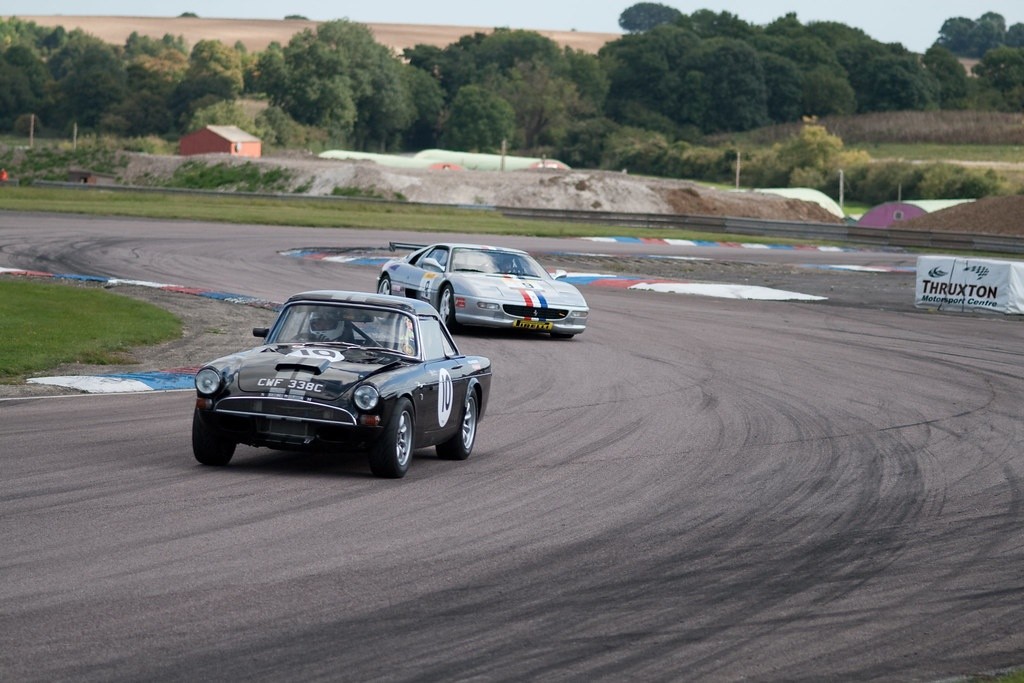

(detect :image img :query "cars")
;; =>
[192,288,492,480]
[375,243,590,340]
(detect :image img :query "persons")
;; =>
[308,308,344,342]
[501,258,524,274]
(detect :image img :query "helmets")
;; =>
[309,312,345,340]
